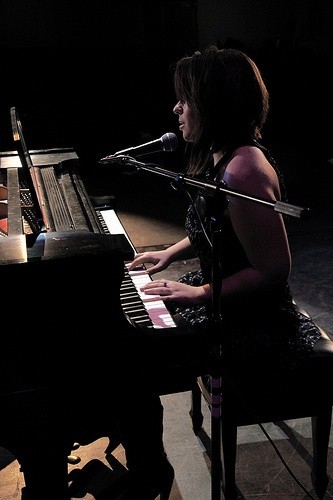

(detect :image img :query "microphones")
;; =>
[100,133,178,162]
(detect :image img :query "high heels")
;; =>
[103,460,174,500]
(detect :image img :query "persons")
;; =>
[96,46,300,500]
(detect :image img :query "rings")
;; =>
[163,281,167,287]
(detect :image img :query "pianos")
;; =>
[0,107,195,499]
